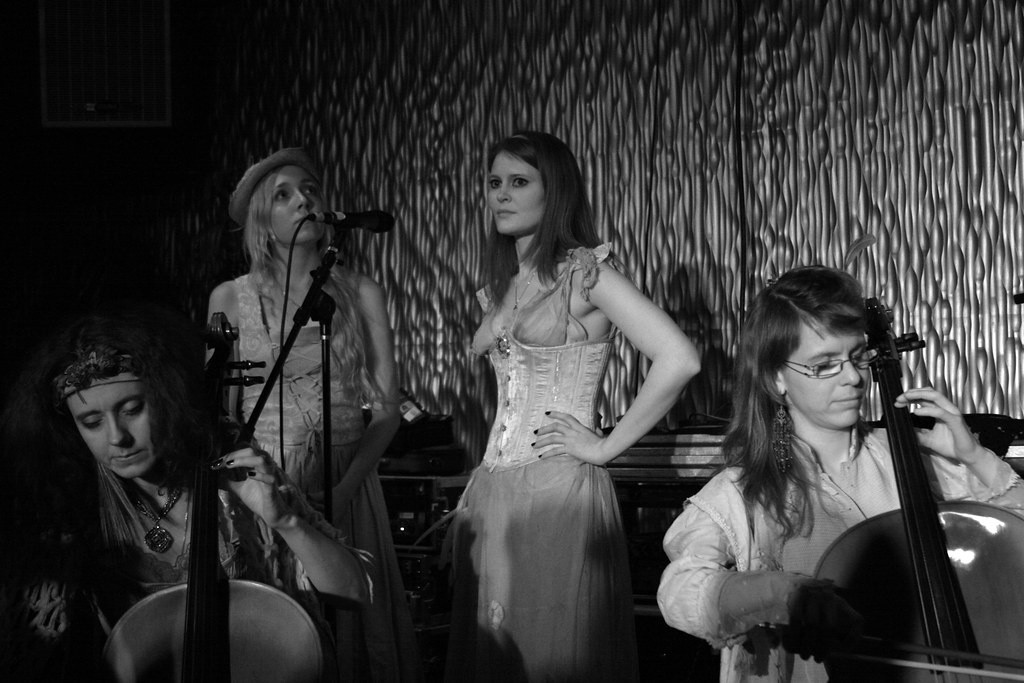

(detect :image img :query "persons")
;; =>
[658,264,1024,683]
[205,148,402,683]
[2,310,387,683]
[470,130,704,683]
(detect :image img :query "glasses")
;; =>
[780,342,871,379]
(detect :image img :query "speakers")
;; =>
[38,0,173,127]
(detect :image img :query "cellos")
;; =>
[803,296,1024,683]
[97,312,341,683]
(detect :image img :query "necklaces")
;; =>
[131,478,185,554]
[268,265,301,307]
[513,269,536,310]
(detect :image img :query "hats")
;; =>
[227,147,322,226]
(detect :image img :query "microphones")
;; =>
[308,209,395,234]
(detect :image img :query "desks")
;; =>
[436,445,1024,491]
